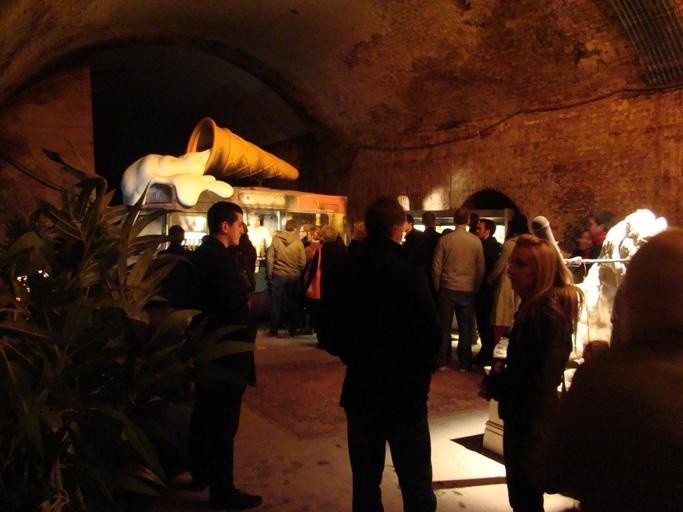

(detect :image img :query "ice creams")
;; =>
[121,117,300,207]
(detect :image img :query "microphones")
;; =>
[531,214,565,266]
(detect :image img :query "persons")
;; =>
[170,201,263,511]
[265,219,366,337]
[474,232,581,511]
[403,207,613,371]
[310,195,449,509]
[236,222,258,387]
[534,229,682,510]
[158,223,191,267]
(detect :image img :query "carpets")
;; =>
[240,351,495,441]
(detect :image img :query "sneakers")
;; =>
[206,486,263,512]
[436,362,486,374]
[265,326,314,338]
